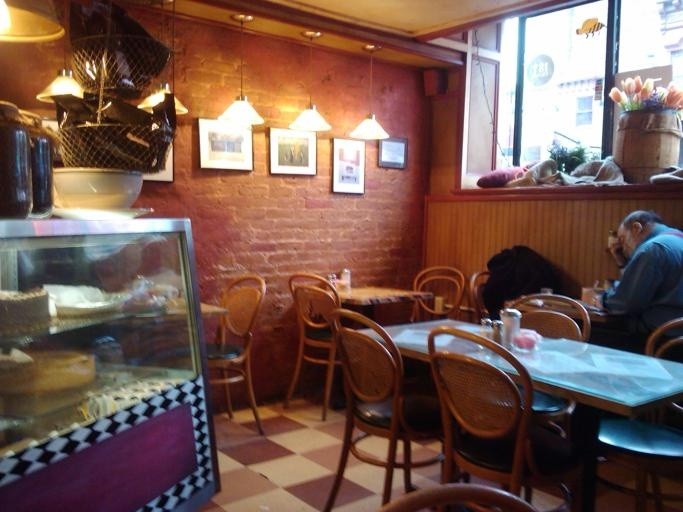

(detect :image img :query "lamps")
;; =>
[137,0,188,117]
[36,0,83,104]
[216,14,265,126]
[0,0,65,43]
[288,31,333,133]
[348,42,390,142]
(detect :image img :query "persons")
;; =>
[591,210,683,364]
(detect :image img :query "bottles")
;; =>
[0,101,54,220]
[500,308,523,350]
[328,269,351,293]
[478,317,504,353]
[541,289,554,295]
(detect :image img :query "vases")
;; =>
[609,109,683,182]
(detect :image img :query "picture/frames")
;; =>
[196,116,255,172]
[267,125,318,177]
[142,138,175,184]
[330,136,367,196]
[377,134,409,170]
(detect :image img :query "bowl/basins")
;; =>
[52,167,144,208]
[51,209,154,220]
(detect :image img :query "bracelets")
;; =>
[615,256,628,269]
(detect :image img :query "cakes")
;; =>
[0,286,48,328]
[0,347,97,403]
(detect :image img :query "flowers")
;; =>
[608,74,683,114]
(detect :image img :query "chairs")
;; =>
[377,483,537,512]
[282,271,353,420]
[427,324,569,512]
[506,293,591,497]
[410,267,466,319]
[596,316,683,512]
[322,307,454,512]
[172,275,266,434]
[468,269,492,324]
